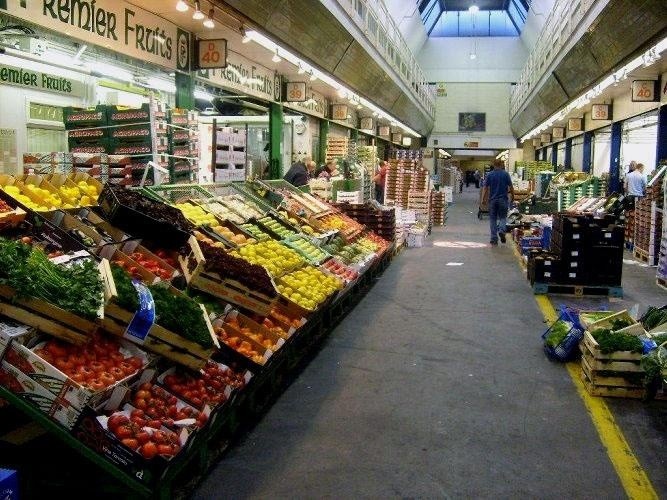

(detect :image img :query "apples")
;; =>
[116,247,184,281]
[193,225,247,251]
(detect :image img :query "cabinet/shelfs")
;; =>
[62,90,201,204]
[327,135,379,202]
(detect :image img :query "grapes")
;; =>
[106,183,187,232]
[178,240,276,305]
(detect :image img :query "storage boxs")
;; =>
[0,358,82,432]
[6,326,157,412]
[155,353,257,435]
[70,379,210,492]
[22,151,133,189]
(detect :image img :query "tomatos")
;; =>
[34,335,246,459]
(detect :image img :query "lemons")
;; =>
[3,181,99,212]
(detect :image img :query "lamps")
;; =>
[518,46,662,144]
[176,0,396,127]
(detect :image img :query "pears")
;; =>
[176,201,219,227]
[230,240,343,311]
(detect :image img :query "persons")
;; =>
[481,158,515,247]
[283,154,337,189]
[372,159,388,205]
[464,160,505,188]
[618,158,667,201]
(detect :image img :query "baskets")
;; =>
[97,180,191,250]
[540,306,585,361]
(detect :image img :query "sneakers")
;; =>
[490,240,498,245]
[499,232,506,243]
